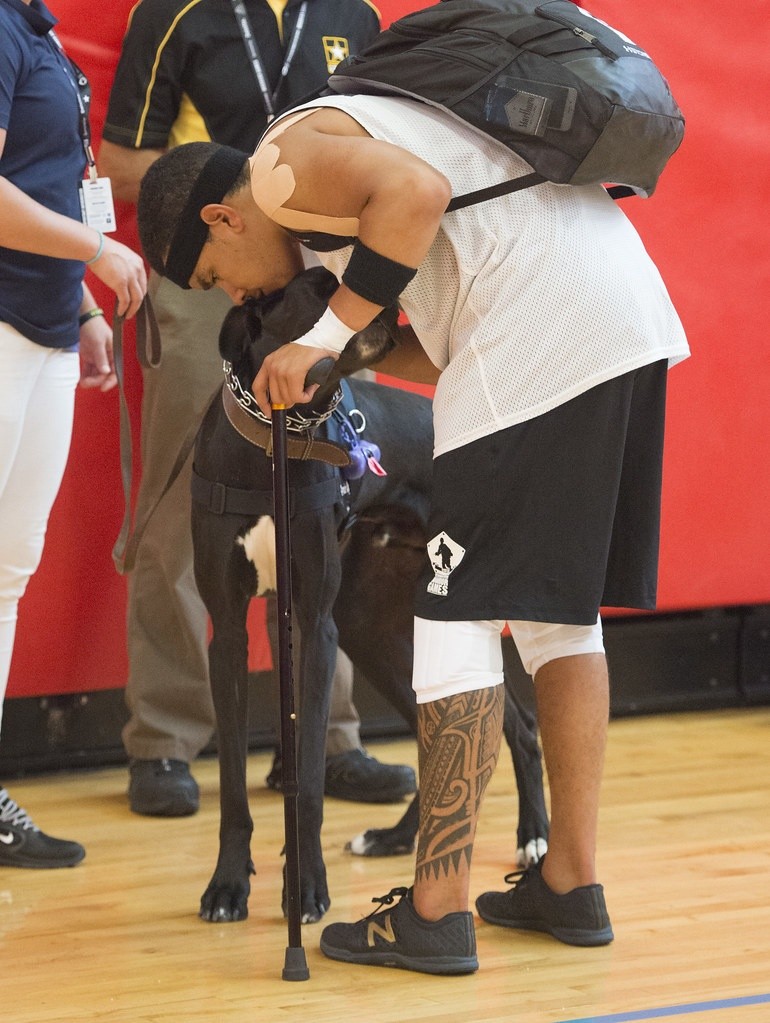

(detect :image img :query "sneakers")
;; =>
[126,759,199,814]
[319,885,478,974]
[267,749,417,802]
[0,786,86,868]
[475,853,614,947]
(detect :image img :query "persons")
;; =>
[0,0,147,867]
[138,94,692,973]
[96,0,417,815]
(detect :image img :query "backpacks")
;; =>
[255,0,687,255]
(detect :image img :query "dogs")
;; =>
[189,265,550,923]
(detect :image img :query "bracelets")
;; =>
[79,308,105,327]
[84,229,105,265]
[291,306,357,353]
